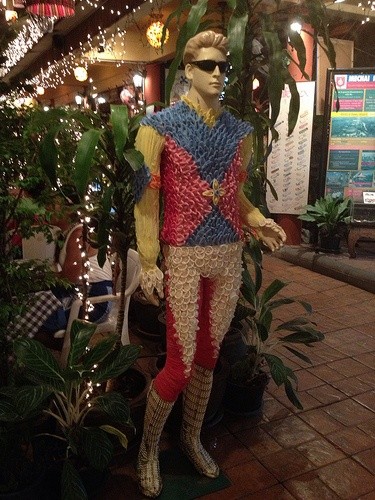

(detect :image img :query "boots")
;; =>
[179,363,220,477]
[136,378,175,498]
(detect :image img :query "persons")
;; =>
[32,182,113,363]
[134,30,286,497]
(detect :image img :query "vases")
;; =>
[22,225,61,261]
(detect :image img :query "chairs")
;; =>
[46,224,143,367]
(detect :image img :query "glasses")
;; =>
[189,60,230,73]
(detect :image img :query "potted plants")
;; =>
[0,250,326,500]
[294,193,355,254]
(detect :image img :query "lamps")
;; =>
[146,0,169,49]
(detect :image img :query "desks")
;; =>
[347,222,375,258]
